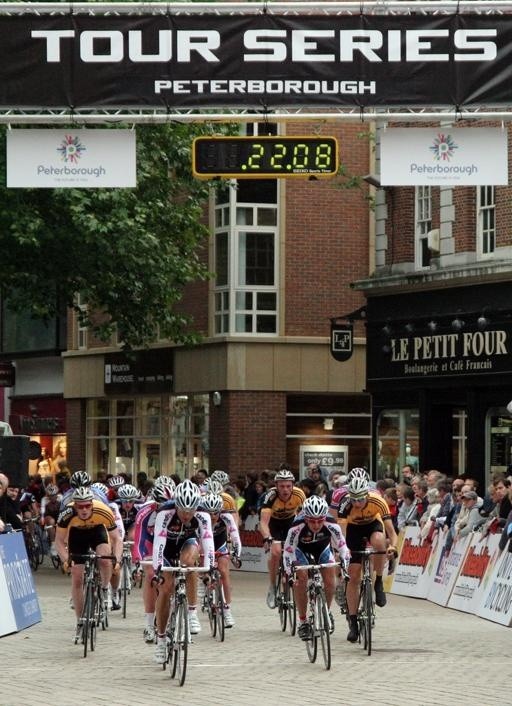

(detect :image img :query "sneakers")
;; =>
[297,622,312,641]
[110,591,121,609]
[346,624,359,641]
[329,611,335,634]
[188,614,202,634]
[266,587,276,609]
[155,644,166,664]
[73,624,84,644]
[223,609,235,627]
[144,624,156,642]
[373,583,387,608]
[49,544,58,557]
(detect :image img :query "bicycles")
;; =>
[25,515,44,565]
[44,524,60,569]
[134,558,213,685]
[288,556,348,670]
[198,549,240,642]
[333,546,399,657]
[63,552,117,658]
[23,524,39,571]
[120,540,134,619]
[261,537,297,637]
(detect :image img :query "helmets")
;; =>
[346,467,371,484]
[69,470,92,487]
[152,484,174,505]
[302,494,330,519]
[108,476,125,487]
[202,494,224,513]
[155,475,176,490]
[45,483,59,496]
[347,476,369,498]
[91,482,110,495]
[117,483,139,499]
[202,477,211,487]
[211,469,231,487]
[174,479,202,512]
[273,469,295,482]
[206,481,224,495]
[72,486,95,502]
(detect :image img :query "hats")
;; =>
[460,491,477,499]
[307,463,319,469]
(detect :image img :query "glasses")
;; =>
[209,511,219,515]
[350,497,365,502]
[308,519,324,524]
[121,498,134,504]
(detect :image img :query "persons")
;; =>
[254,480,267,515]
[257,469,308,610]
[196,493,241,629]
[115,484,146,541]
[395,465,488,542]
[196,469,207,487]
[0,473,24,535]
[493,477,511,528]
[338,477,399,644]
[153,478,216,666]
[16,485,40,532]
[330,468,383,521]
[92,482,126,541]
[282,495,350,639]
[204,480,240,524]
[60,471,108,511]
[131,475,176,644]
[55,487,123,627]
[242,470,259,500]
[211,470,239,500]
[7,481,19,502]
[488,483,500,520]
[44,485,61,558]
